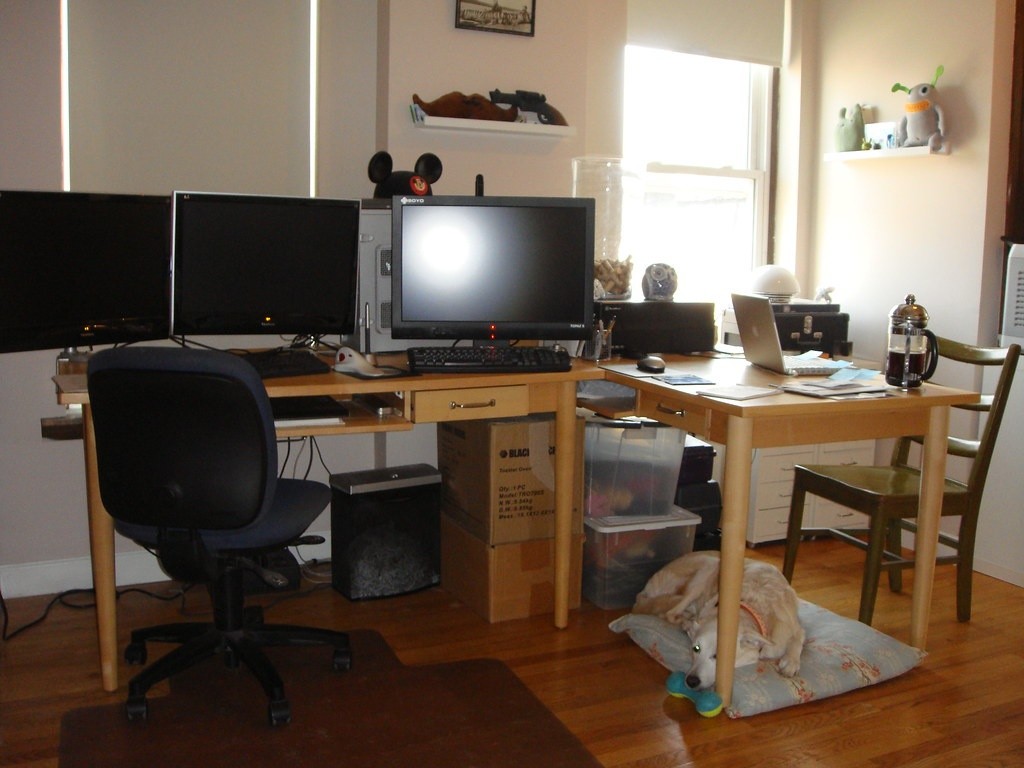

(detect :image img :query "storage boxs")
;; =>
[438,415,723,623]
[329,464,444,600]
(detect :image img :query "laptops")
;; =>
[731,293,844,375]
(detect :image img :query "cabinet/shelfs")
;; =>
[746,439,875,549]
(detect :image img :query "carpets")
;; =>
[58,660,608,768]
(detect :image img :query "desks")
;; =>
[56,363,605,692]
[574,356,982,708]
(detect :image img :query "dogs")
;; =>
[631,549,805,689]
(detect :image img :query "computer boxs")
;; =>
[328,463,442,602]
[340,199,510,354]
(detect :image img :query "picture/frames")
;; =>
[455,0,535,37]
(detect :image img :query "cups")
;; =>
[884,295,940,389]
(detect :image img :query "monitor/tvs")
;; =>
[391,195,595,341]
[0,190,172,354]
[171,190,362,335]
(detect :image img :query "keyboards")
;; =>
[406,346,572,373]
[229,350,330,380]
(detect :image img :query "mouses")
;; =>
[333,347,384,377]
[637,356,666,374]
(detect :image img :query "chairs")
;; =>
[86,346,355,728]
[785,334,1021,627]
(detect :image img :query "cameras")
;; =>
[533,344,567,352]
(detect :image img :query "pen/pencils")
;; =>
[598,316,617,350]
